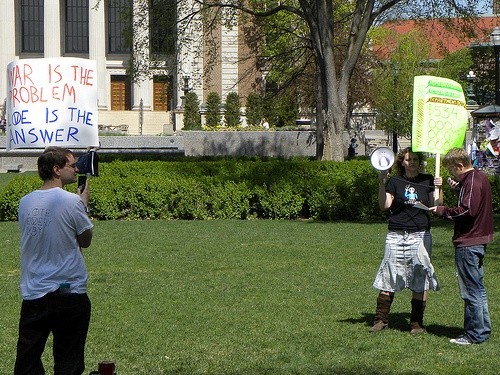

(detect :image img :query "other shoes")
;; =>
[450,338,471,344]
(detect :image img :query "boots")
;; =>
[410,297,426,333]
[369,293,394,333]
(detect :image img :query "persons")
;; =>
[348,139,357,157]
[86,141,101,176]
[429,147,493,344]
[368,147,443,334]
[14,146,94,375]
[261,118,269,129]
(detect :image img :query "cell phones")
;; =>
[77,176,87,193]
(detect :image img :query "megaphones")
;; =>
[369,146,395,177]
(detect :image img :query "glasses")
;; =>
[64,163,75,168]
